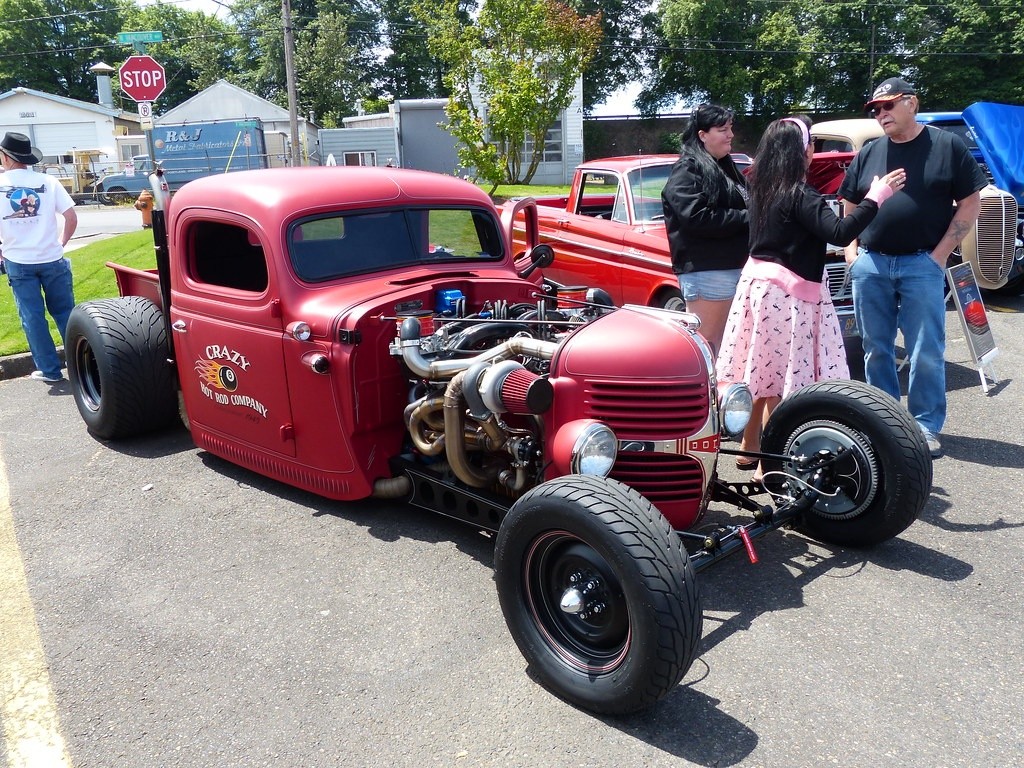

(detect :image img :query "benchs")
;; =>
[577,203,663,221]
[193,209,423,292]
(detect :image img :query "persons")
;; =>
[661,105,751,364]
[0,132,77,382]
[714,113,906,483]
[836,77,989,456]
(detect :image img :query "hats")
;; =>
[0,132,43,164]
[864,77,916,112]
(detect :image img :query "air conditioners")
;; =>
[116,125,129,136]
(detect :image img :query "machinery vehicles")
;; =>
[38,148,120,208]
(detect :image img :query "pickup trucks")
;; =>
[489,150,863,374]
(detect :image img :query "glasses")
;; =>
[870,98,908,116]
[692,103,707,125]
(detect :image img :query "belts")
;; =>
[858,244,934,256]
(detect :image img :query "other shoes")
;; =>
[31,370,64,382]
[923,431,942,457]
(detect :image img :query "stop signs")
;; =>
[119,56,168,102]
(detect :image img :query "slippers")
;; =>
[736,459,759,471]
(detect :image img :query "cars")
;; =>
[809,118,1019,291]
[908,102,1024,283]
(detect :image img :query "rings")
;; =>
[896,181,899,185]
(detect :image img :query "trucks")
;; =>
[100,117,269,201]
